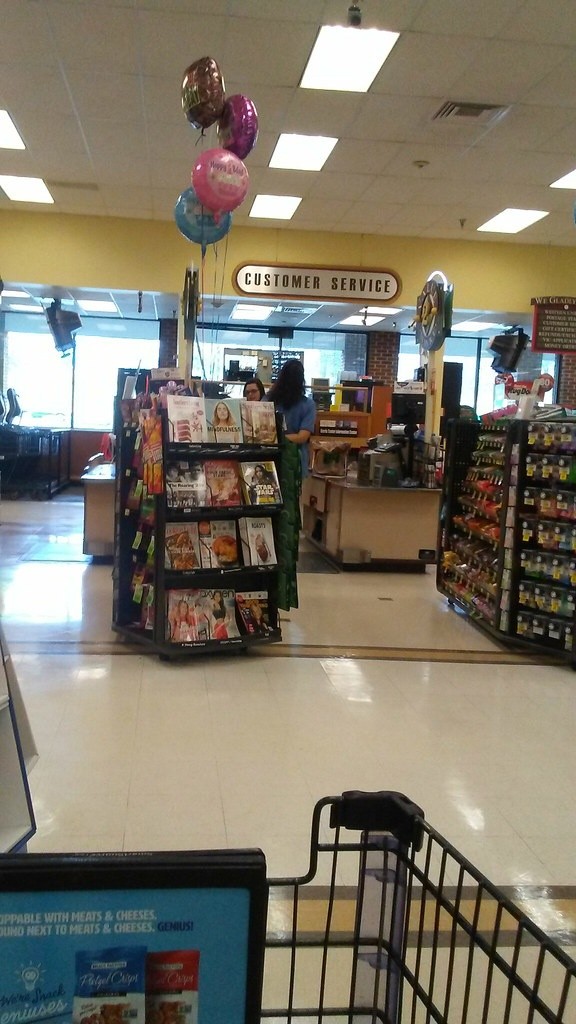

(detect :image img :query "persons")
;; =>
[255,416,276,443]
[263,359,316,478]
[209,402,239,443]
[166,463,180,507]
[209,591,229,639]
[247,603,270,634]
[254,465,280,503]
[172,600,197,641]
[242,378,265,401]
[185,462,205,506]
[216,468,239,506]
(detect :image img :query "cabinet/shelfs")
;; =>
[191,378,392,448]
[435,416,576,671]
[301,468,442,571]
[80,452,116,562]
[114,367,288,660]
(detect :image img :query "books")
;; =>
[165,395,283,641]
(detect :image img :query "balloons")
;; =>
[175,187,232,257]
[216,94,259,160]
[181,56,226,129]
[191,148,249,224]
